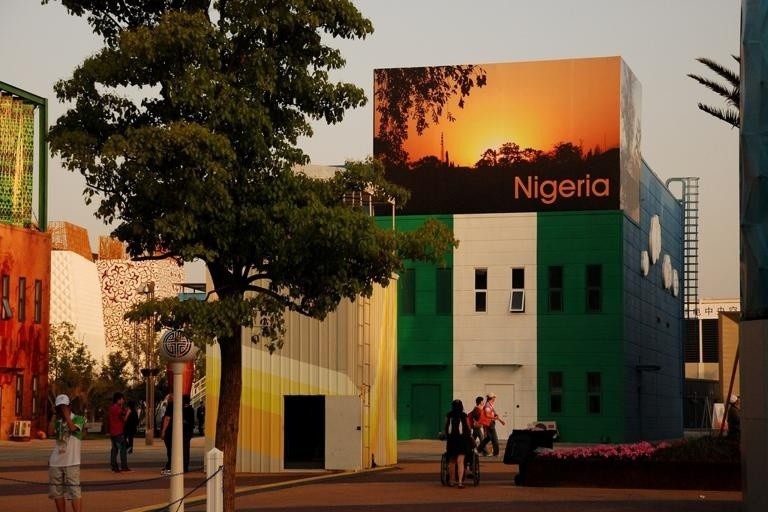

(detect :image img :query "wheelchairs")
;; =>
[440,450,482,487]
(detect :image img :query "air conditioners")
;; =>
[13,420,32,438]
[533,421,558,439]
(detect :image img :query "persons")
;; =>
[197,401,205,436]
[183,395,196,473]
[473,392,507,457]
[107,392,139,473]
[445,400,470,488]
[728,394,741,438]
[160,390,173,475]
[48,394,85,512]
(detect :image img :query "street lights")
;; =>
[137,280,159,446]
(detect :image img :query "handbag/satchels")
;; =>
[478,406,492,427]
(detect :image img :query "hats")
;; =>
[55,394,70,407]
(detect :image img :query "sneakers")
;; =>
[161,470,171,475]
[448,483,465,489]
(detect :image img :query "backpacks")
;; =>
[466,411,474,429]
